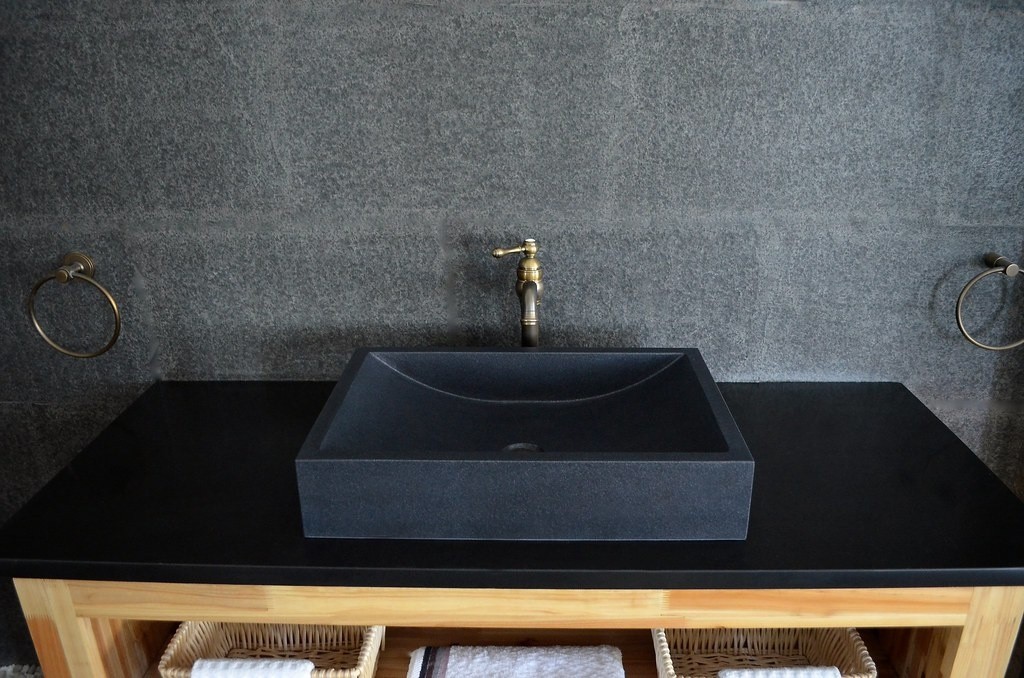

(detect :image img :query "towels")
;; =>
[189,657,314,678]
[716,664,842,678]
[405,641,625,678]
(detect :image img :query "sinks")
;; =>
[294,347,756,541]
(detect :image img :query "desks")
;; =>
[10,579,1024,678]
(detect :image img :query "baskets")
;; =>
[157,620,383,678]
[650,628,877,678]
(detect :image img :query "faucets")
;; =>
[493,238,545,348]
